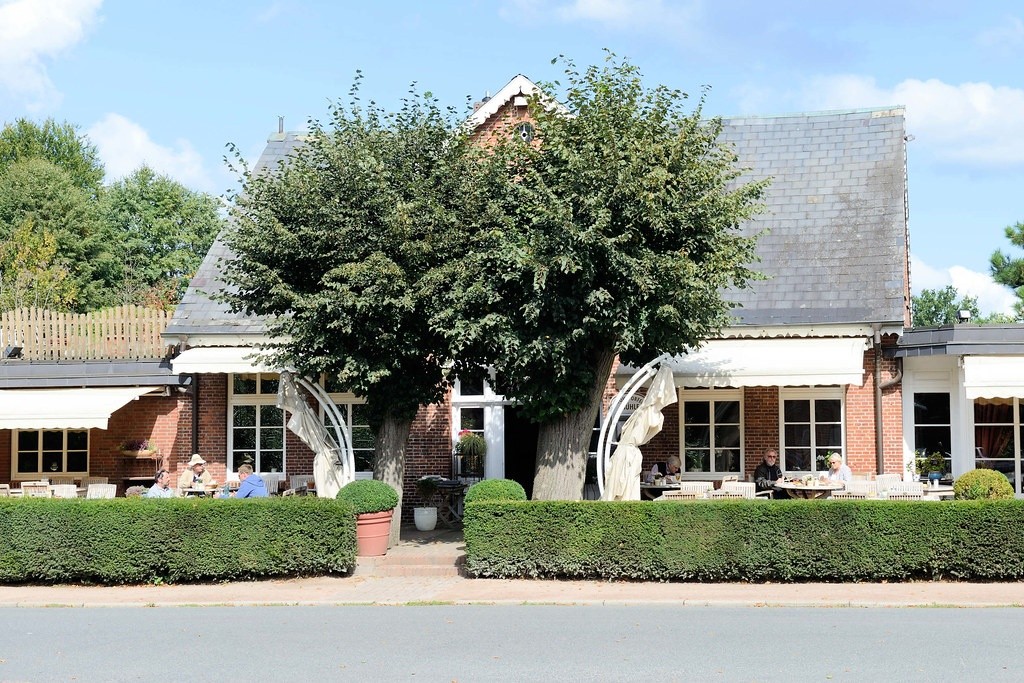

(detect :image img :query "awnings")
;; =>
[963,356,1024,401]
[169,347,303,374]
[612,337,874,388]
[0,386,177,432]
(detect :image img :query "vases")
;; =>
[933,479,939,489]
[122,450,156,457]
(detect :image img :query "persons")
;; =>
[816,452,852,499]
[146,470,175,498]
[230,464,270,498]
[178,454,216,498]
[646,456,682,497]
[753,448,790,499]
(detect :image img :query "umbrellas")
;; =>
[597,353,678,502]
[276,369,355,499]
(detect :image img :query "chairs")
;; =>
[282,486,308,497]
[721,481,755,499]
[0,484,10,497]
[709,492,744,499]
[51,476,74,485]
[830,473,924,501]
[682,483,709,493]
[748,474,773,499]
[642,471,650,482]
[48,484,77,498]
[80,476,108,488]
[662,490,697,500]
[20,481,49,497]
[86,484,117,499]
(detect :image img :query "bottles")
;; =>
[662,477,666,486]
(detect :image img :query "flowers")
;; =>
[118,439,155,451]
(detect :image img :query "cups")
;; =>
[934,479,939,488]
[655,480,660,485]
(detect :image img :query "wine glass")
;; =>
[675,473,681,484]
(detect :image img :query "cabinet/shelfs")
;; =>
[119,454,164,484]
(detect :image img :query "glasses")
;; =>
[767,455,777,459]
[830,460,838,465]
[238,472,243,478]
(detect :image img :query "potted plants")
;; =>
[807,476,814,486]
[905,449,925,482]
[336,479,399,556]
[455,432,487,473]
[413,477,438,532]
[685,441,707,472]
[197,479,205,490]
[925,451,945,479]
[655,474,661,486]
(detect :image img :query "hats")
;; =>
[187,454,206,466]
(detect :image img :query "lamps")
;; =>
[5,346,23,357]
[955,308,971,324]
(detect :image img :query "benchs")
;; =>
[774,482,843,499]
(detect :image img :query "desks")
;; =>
[653,493,709,501]
[307,489,317,497]
[413,482,468,529]
[640,484,681,500]
[923,485,953,492]
[10,488,86,496]
[182,487,238,498]
